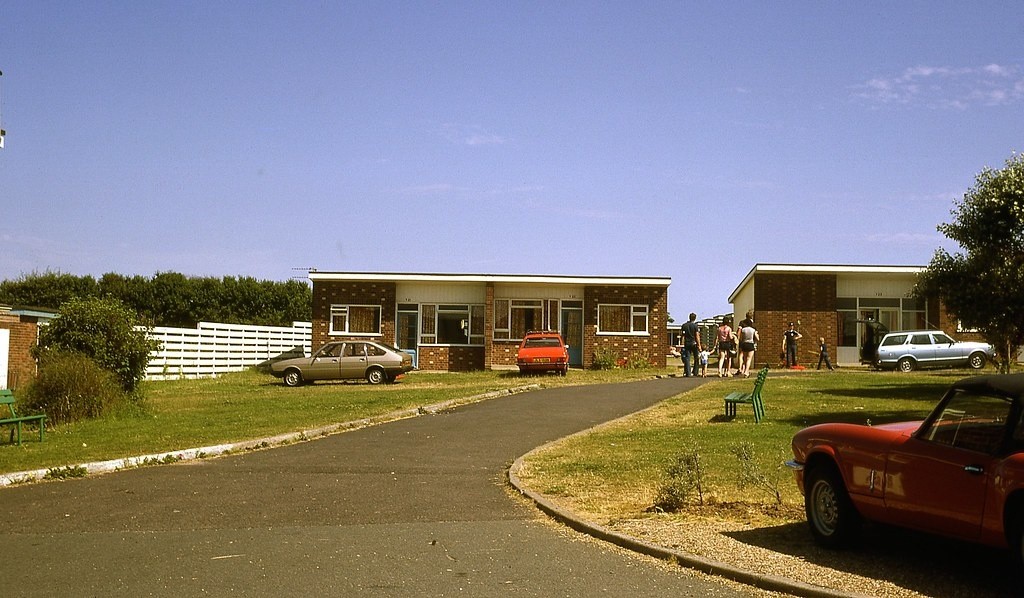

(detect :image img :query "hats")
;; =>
[789,322,794,326]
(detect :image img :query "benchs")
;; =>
[0,388,47,446]
[724,366,770,422]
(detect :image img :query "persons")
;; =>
[680,313,702,376]
[714,316,735,378]
[698,343,715,378]
[739,320,759,378]
[782,322,802,369]
[817,337,834,370]
[735,313,757,374]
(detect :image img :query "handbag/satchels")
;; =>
[728,350,737,358]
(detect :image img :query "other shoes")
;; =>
[717,373,722,378]
[737,369,742,375]
[724,371,727,376]
[743,372,750,378]
[727,373,734,377]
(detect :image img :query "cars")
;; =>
[515,332,570,377]
[854,320,995,373]
[269,341,411,387]
[783,372,1023,574]
